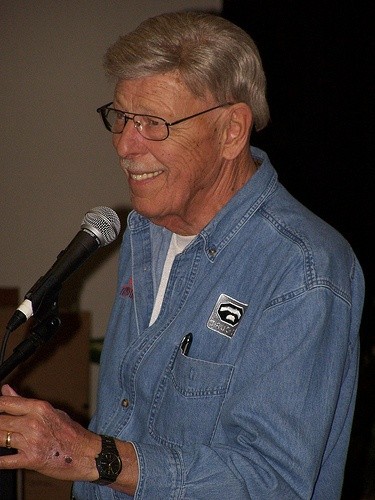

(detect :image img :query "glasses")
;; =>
[97,102,234,142]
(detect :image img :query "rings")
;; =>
[6,431,11,448]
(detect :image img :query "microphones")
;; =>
[6,205,121,331]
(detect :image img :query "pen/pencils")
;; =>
[170,331,194,372]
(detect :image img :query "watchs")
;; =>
[90,435,122,487]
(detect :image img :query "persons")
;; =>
[0,9,366,500]
